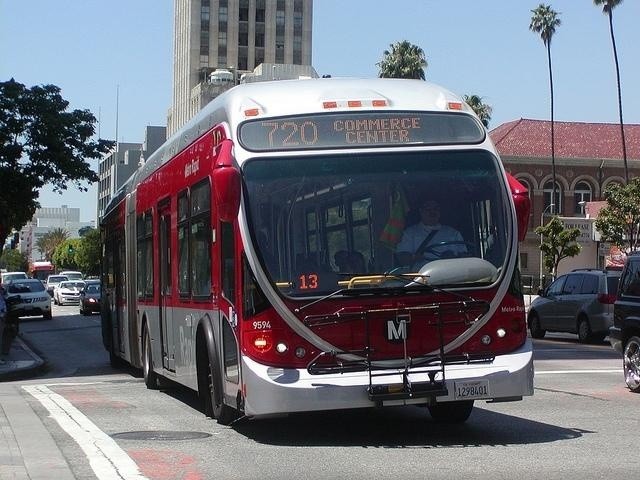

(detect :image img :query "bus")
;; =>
[97,75,538,435]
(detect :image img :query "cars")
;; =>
[0,262,102,355]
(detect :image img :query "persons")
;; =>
[334,250,349,274]
[392,194,471,268]
[0,290,8,365]
[349,251,368,275]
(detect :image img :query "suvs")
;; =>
[527,266,623,343]
[607,251,640,393]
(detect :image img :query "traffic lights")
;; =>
[68,244,73,253]
